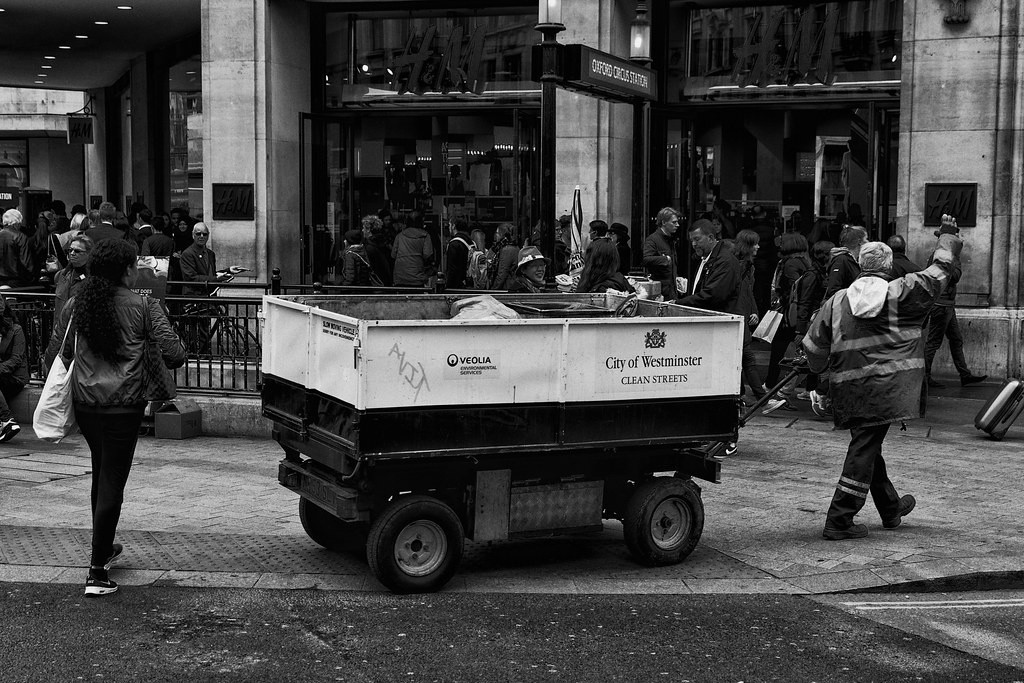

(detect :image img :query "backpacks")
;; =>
[788,271,819,327]
[447,237,489,290]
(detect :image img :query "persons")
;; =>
[0,195,242,443]
[802,214,964,540]
[45,236,187,594]
[328,183,988,414]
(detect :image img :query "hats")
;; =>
[344,229,364,246]
[514,246,551,275]
[607,223,630,241]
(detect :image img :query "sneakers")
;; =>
[714,445,737,459]
[882,495,916,530]
[926,374,946,389]
[960,373,988,386]
[739,383,834,417]
[84,573,117,596]
[0,418,21,442]
[103,540,124,571]
[823,522,868,541]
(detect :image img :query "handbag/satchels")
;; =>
[141,297,177,400]
[345,251,385,290]
[45,233,59,272]
[32,353,77,444]
[751,310,784,344]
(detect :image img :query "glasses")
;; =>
[556,228,562,233]
[529,262,546,268]
[68,247,87,253]
[193,232,209,236]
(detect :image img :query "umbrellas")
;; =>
[567,185,585,272]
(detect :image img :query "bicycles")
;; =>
[170,276,264,361]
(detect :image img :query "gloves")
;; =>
[812,373,830,394]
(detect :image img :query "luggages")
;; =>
[973,378,1024,441]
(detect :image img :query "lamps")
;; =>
[629,0,654,67]
